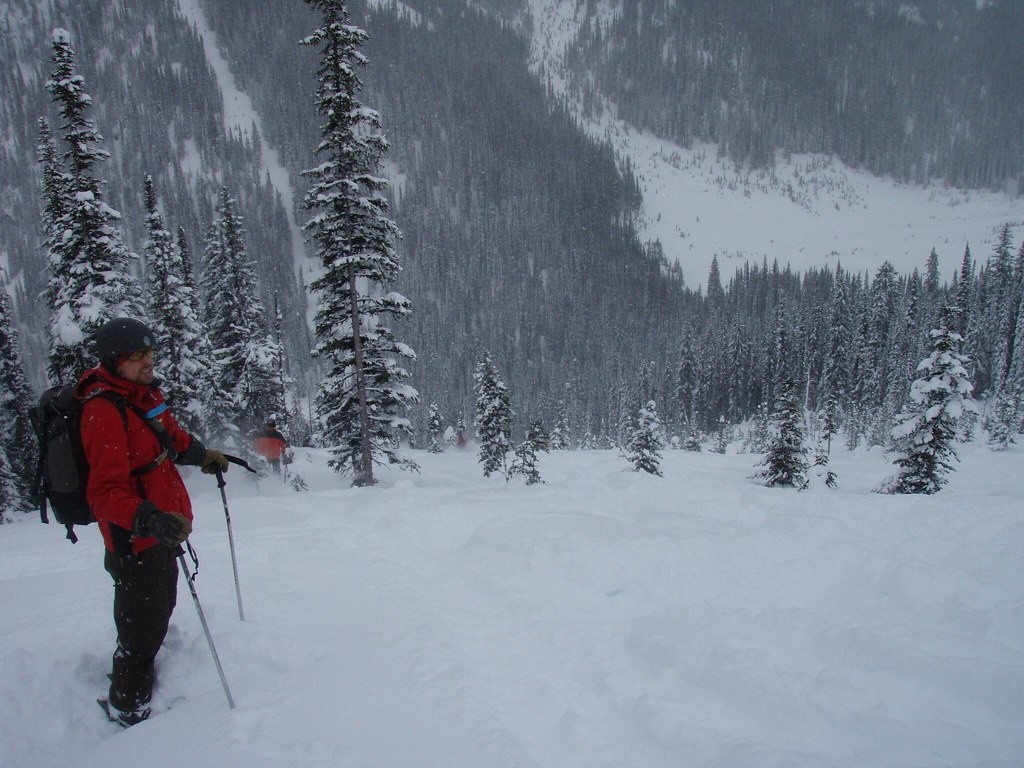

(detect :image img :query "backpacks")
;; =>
[26,374,126,543]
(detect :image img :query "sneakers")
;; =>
[104,685,157,727]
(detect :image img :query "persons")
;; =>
[72,315,228,729]
[255,418,288,478]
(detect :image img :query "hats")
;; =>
[263,418,277,425]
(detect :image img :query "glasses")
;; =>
[118,347,157,361]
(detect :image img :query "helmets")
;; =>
[95,317,157,359]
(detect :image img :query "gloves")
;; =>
[133,500,195,548]
[201,450,229,474]
